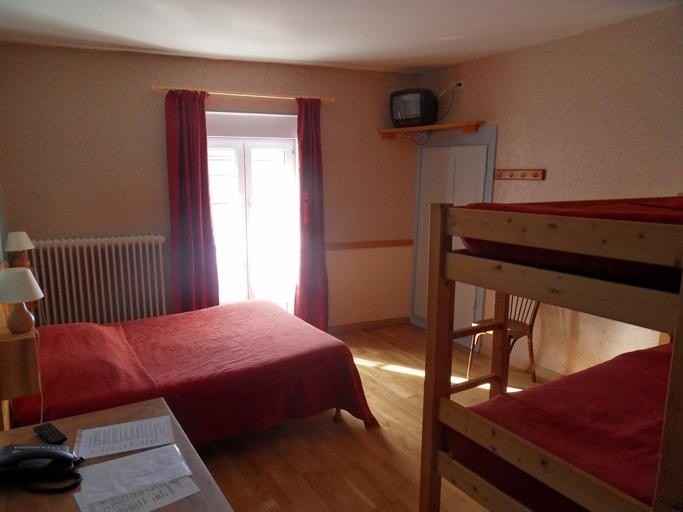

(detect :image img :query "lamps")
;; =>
[0,267,45,334]
[3,232,36,267]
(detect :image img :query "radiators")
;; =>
[27,235,170,328]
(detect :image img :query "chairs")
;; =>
[464,293,540,383]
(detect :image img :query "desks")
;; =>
[0,395,234,512]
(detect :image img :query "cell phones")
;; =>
[34,422,67,444]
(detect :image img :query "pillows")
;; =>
[11,321,158,427]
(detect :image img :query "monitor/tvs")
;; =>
[389,88,439,127]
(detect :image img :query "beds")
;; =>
[0,261,380,447]
[418,200,683,512]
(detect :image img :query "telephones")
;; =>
[0,444,77,482]
